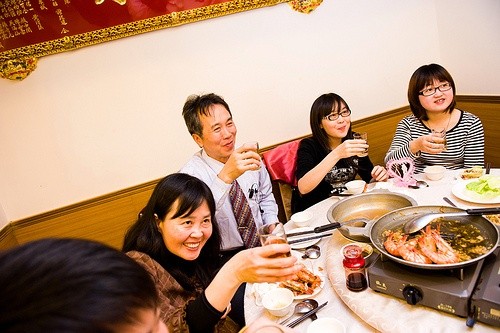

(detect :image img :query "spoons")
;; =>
[280,299,318,324]
[299,249,321,259]
[411,175,428,187]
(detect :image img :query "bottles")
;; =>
[342,245,368,292]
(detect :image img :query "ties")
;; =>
[229,178,262,248]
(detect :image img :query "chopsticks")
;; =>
[408,185,420,189]
[285,230,333,244]
[287,301,328,326]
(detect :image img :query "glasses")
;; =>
[322,109,351,121]
[418,82,452,96]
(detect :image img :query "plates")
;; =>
[285,226,322,249]
[452,175,500,204]
[365,182,394,192]
[278,275,325,300]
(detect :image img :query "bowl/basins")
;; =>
[262,289,294,317]
[340,242,373,266]
[345,180,366,194]
[424,165,446,180]
[291,211,314,226]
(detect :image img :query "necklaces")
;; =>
[427,114,450,132]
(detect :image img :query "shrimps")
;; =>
[382,224,459,265]
[279,266,322,296]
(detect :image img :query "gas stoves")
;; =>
[365,246,500,329]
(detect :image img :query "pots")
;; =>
[363,205,499,268]
[325,190,418,241]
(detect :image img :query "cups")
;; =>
[243,142,262,170]
[431,126,447,152]
[258,222,292,258]
[353,131,368,157]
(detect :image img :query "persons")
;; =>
[384,64,485,174]
[0,237,170,333]
[292,93,389,214]
[122,173,302,333]
[179,93,279,326]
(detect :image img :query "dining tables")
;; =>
[243,168,500,333]
